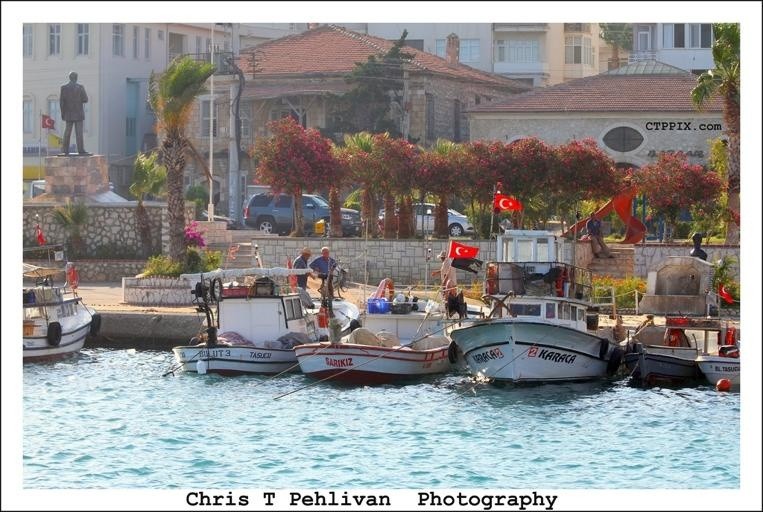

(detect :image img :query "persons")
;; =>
[60,71,88,155]
[587,211,615,258]
[432,251,458,309]
[691,232,707,261]
[293,246,338,299]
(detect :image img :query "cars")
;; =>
[245,190,364,239]
[376,202,474,237]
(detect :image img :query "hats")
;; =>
[300,247,313,254]
[437,251,446,258]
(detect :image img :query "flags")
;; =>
[494,193,522,211]
[42,115,54,129]
[448,240,483,274]
[719,283,733,303]
[36,223,46,245]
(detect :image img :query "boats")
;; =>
[451,225,620,384]
[625,339,705,384]
[696,352,739,390]
[172,274,359,373]
[22,263,93,360]
[292,311,454,378]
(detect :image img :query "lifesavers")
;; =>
[381,278,394,300]
[487,263,496,295]
[318,306,330,338]
[556,266,571,298]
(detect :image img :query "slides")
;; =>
[612,187,646,244]
[560,201,611,238]
